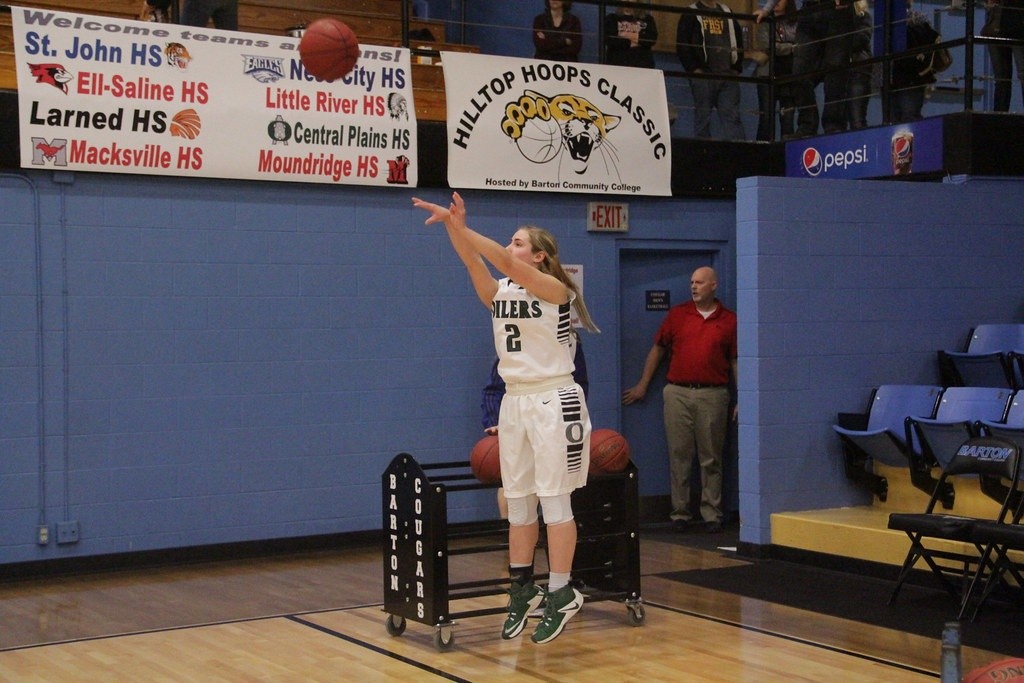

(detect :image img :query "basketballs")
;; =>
[299,19,360,82]
[589,428,631,475]
[470,436,503,487]
[963,657,1024,683]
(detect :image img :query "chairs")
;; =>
[834,322,1024,623]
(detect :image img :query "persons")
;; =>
[604,0,658,69]
[411,191,600,644]
[622,266,738,533]
[481,327,588,543]
[746,0,953,143]
[532,0,584,62]
[974,0,1024,112]
[676,0,744,141]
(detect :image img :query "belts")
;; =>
[665,378,732,390]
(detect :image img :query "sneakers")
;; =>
[703,519,723,533]
[501,584,547,640]
[530,583,585,644]
[666,517,689,532]
